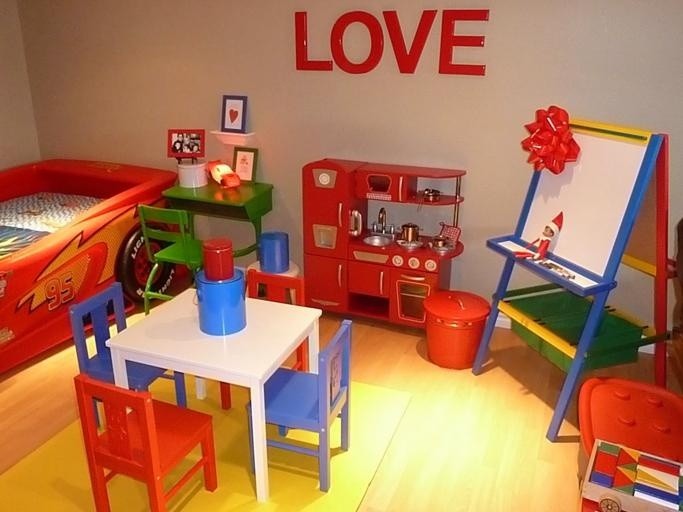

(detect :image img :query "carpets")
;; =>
[0,370,414,512]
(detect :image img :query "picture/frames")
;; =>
[219,94,247,133]
[165,127,206,165]
[231,144,259,186]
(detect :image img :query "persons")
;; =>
[174,133,200,153]
[512,211,563,262]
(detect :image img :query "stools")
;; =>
[242,259,301,304]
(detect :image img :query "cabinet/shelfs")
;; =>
[298,157,465,332]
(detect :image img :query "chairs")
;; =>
[134,201,204,316]
[219,265,307,411]
[72,370,219,511]
[67,278,186,431]
[243,317,355,491]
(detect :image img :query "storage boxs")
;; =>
[576,375,683,494]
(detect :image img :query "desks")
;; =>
[160,162,273,260]
[103,286,322,502]
